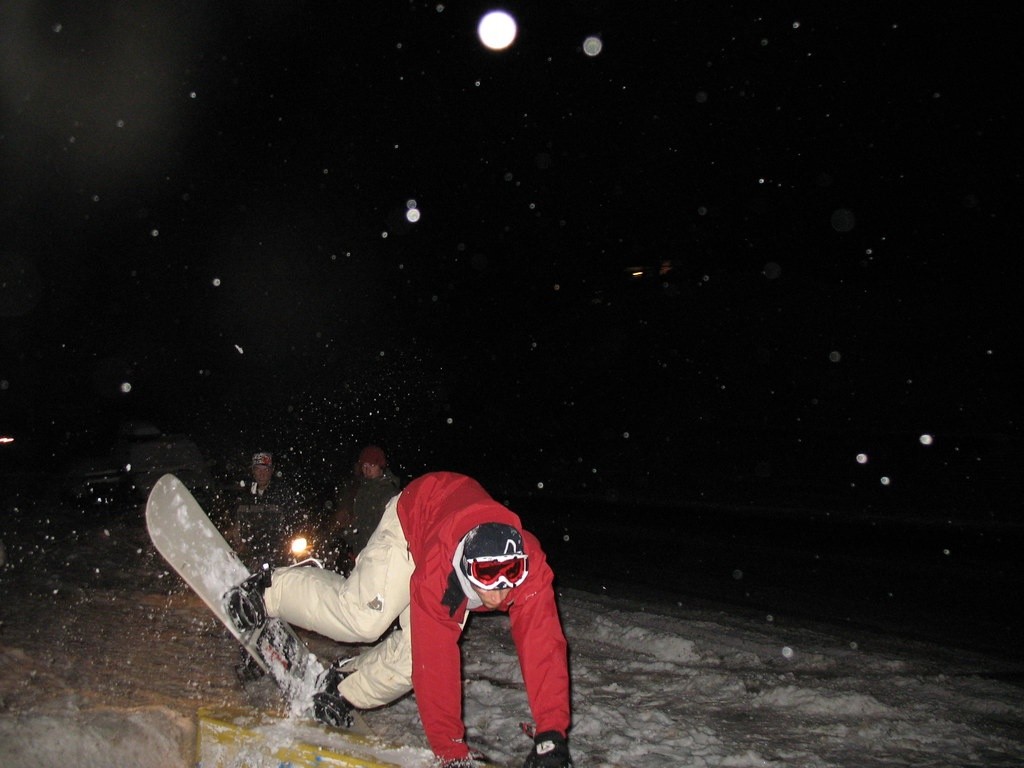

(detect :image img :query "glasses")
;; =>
[253,467,272,473]
[463,554,529,590]
[361,463,377,471]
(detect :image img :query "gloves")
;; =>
[523,731,572,768]
[442,751,493,768]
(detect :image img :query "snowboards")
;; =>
[145,473,373,738]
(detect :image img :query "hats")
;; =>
[251,453,273,469]
[464,523,525,588]
[358,447,385,466]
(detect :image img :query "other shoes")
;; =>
[229,567,272,632]
[317,657,355,727]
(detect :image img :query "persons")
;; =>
[224,473,570,768]
[223,446,404,577]
